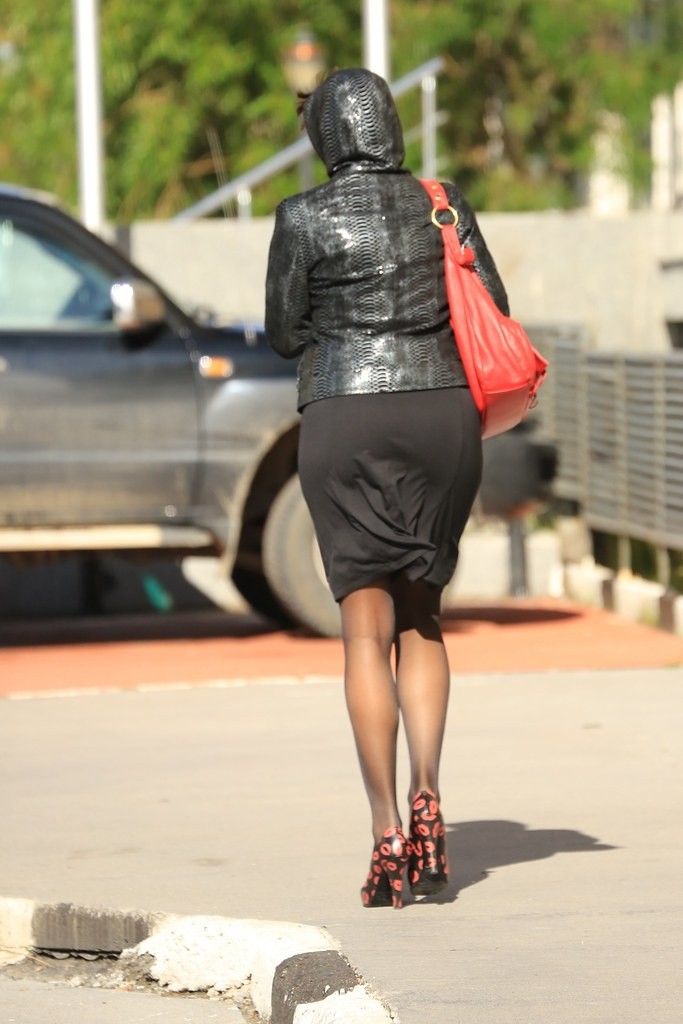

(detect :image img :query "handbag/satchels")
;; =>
[417,178,549,440]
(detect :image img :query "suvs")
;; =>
[0,181,460,642]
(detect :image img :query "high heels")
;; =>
[408,790,449,895]
[361,827,413,909]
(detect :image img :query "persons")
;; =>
[265,66,510,909]
